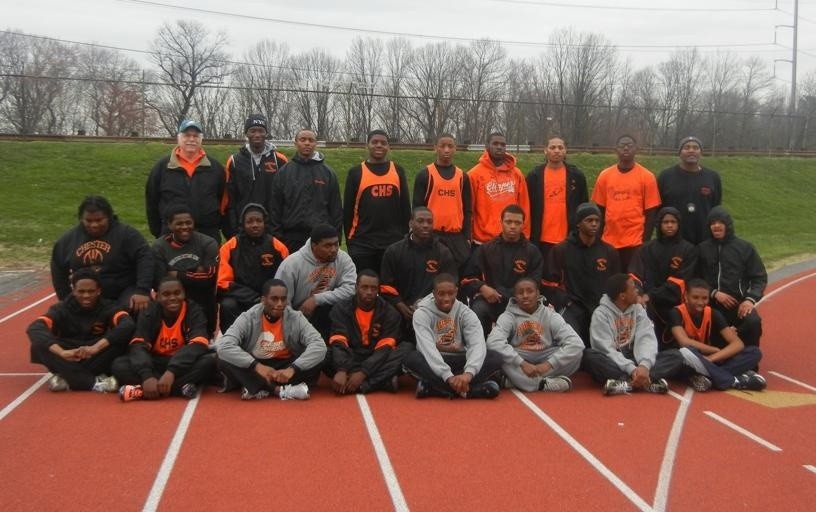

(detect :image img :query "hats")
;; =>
[575,203,602,224]
[178,119,206,134]
[678,137,705,156]
[71,268,101,285]
[244,114,269,134]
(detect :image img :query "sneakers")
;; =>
[241,385,272,401]
[544,376,574,393]
[49,374,70,393]
[500,374,515,392]
[278,384,313,401]
[181,382,200,401]
[647,379,671,396]
[121,384,145,404]
[378,374,402,392]
[415,379,447,398]
[603,379,634,395]
[465,381,501,400]
[93,376,119,392]
[734,369,768,392]
[684,375,715,393]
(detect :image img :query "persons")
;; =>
[467,131,531,254]
[525,137,589,259]
[274,222,358,338]
[486,277,586,393]
[220,113,288,243]
[406,272,505,399]
[546,202,623,323]
[458,204,545,337]
[655,136,722,243]
[271,129,341,257]
[150,205,221,335]
[378,206,458,339]
[696,205,768,371]
[144,117,226,240]
[665,277,767,391]
[218,278,328,401]
[626,206,701,319]
[343,130,412,271]
[26,269,135,392]
[49,195,155,321]
[414,133,472,262]
[592,134,663,274]
[320,269,415,395]
[581,272,684,396]
[112,275,217,403]
[217,203,291,335]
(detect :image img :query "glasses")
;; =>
[618,143,635,147]
